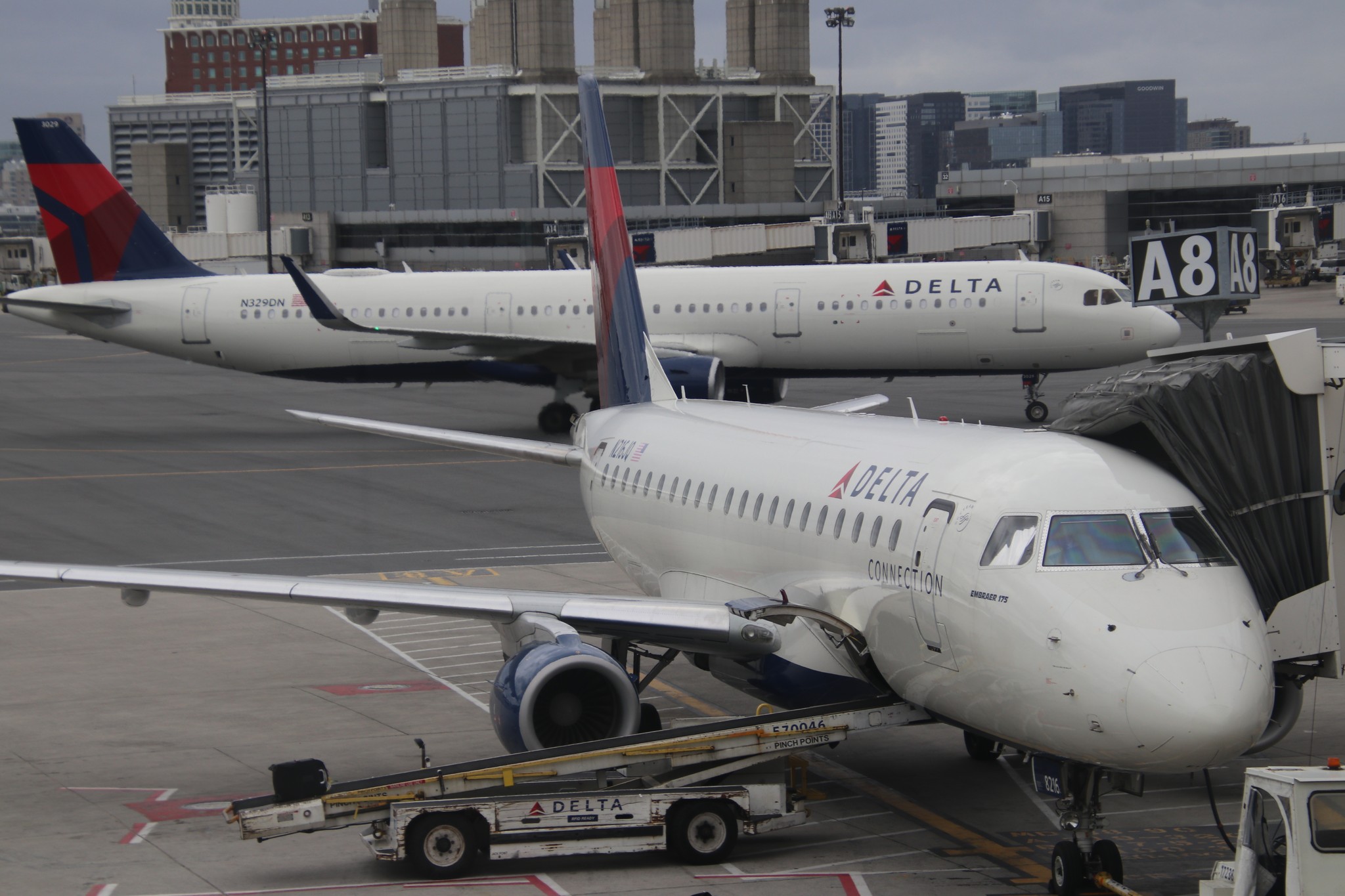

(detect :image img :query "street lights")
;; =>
[823,6,856,211]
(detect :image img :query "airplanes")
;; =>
[0,1,1189,436]
[0,77,1345,896]
